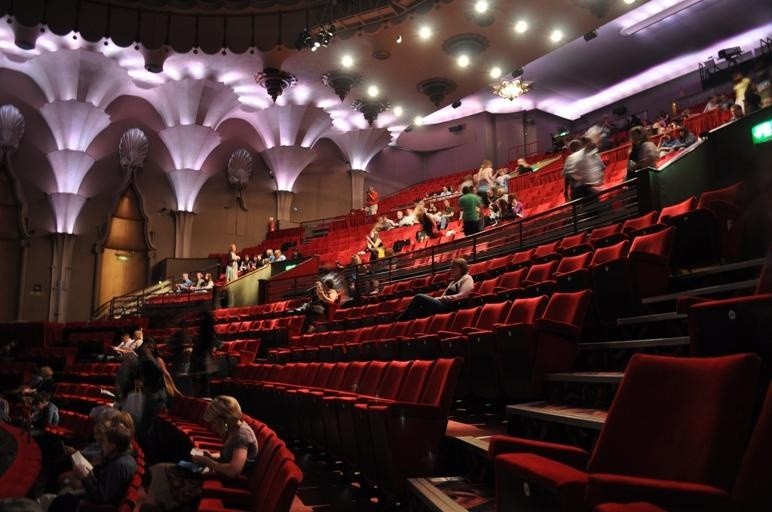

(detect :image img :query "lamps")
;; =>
[301,24,337,52]
[487,75,537,102]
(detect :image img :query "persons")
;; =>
[167,242,302,295]
[296,156,537,334]
[0,307,262,512]
[558,69,772,230]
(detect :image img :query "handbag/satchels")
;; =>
[172,461,206,480]
[125,385,145,423]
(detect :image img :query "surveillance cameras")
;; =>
[718,46,742,59]
